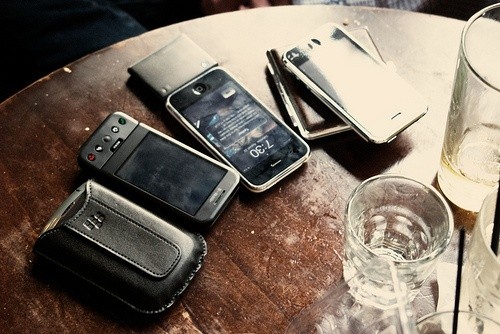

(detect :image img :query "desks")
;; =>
[0,5,500,334]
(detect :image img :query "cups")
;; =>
[412,309,499,334]
[342,174,454,309]
[460,186,499,334]
[437,0,500,212]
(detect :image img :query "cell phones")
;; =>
[165,66,312,193]
[282,24,428,144]
[78,111,241,230]
